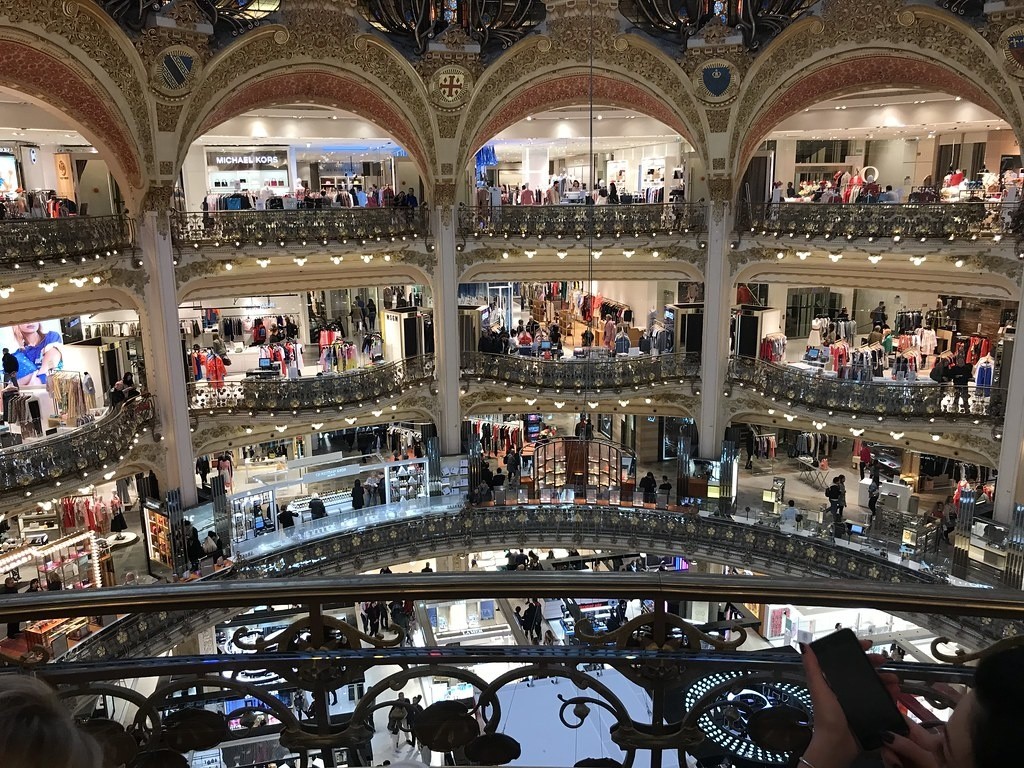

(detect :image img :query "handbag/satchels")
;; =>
[869,492,879,498]
[949,512,957,520]
[503,456,508,464]
[870,310,875,319]
[813,457,819,468]
[823,340,829,347]
[222,353,231,367]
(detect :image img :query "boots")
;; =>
[945,538,952,545]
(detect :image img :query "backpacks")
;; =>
[825,488,829,497]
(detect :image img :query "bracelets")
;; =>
[799,757,814,768]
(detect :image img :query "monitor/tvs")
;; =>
[541,341,551,350]
[255,515,265,530]
[808,349,819,358]
[851,525,863,534]
[259,358,271,370]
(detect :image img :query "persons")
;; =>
[478,413,672,508]
[94,489,127,542]
[276,469,386,533]
[769,441,993,552]
[744,431,754,469]
[243,544,907,768]
[788,182,795,196]
[304,183,418,209]
[0,321,63,388]
[110,372,141,409]
[794,634,1024,768]
[212,295,382,368]
[864,175,879,204]
[499,179,620,206]
[0,188,47,220]
[2,572,138,640]
[478,312,617,368]
[2,348,20,389]
[883,185,900,202]
[173,519,224,583]
[824,301,994,416]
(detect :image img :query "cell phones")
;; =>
[808,627,910,753]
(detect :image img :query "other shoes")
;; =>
[190,565,198,573]
[393,752,399,758]
[745,465,752,469]
[409,742,415,747]
[396,749,402,753]
[406,740,411,743]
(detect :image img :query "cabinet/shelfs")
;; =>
[0,156,1023,767]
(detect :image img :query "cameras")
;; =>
[403,195,407,197]
